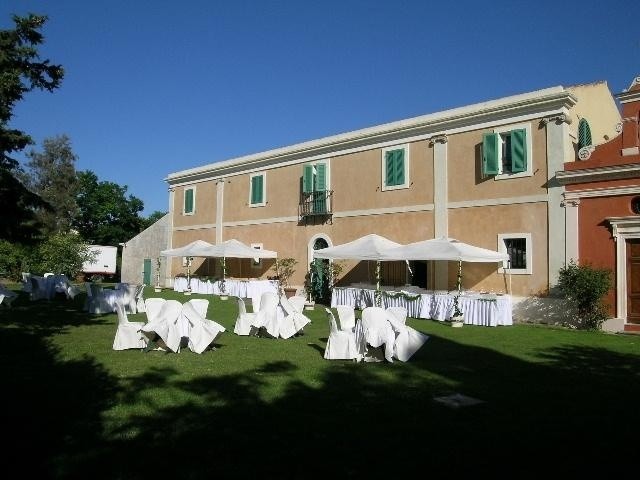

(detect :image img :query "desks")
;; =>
[330,284,513,327]
[173,276,280,299]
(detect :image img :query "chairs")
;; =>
[233,289,432,365]
[0,272,229,355]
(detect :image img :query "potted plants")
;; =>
[448,295,465,328]
[270,256,299,299]
[183,256,193,296]
[302,261,318,311]
[154,257,162,293]
[217,278,229,301]
